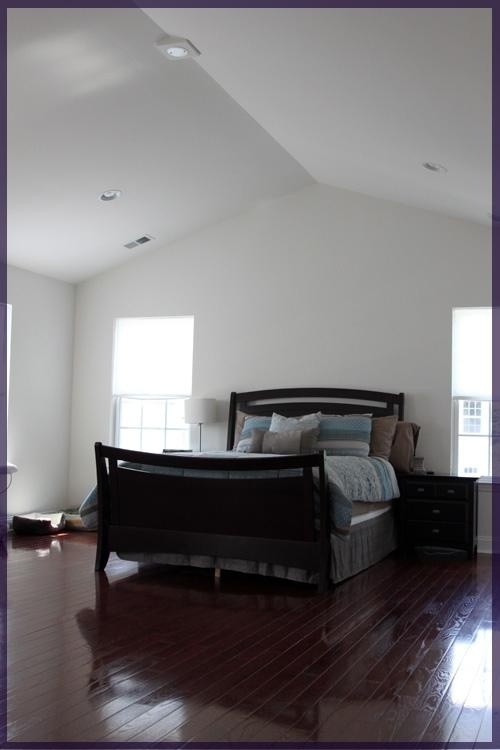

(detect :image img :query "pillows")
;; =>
[224,406,422,477]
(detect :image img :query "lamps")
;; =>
[183,394,221,453]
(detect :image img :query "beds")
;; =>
[79,386,423,611]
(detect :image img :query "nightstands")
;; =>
[397,470,483,562]
